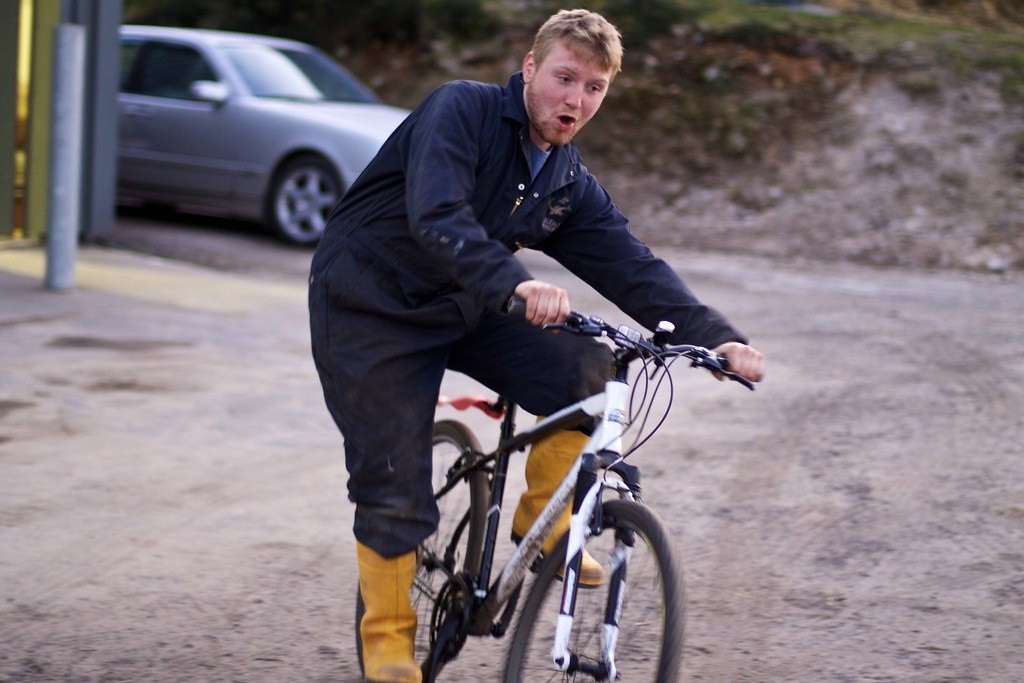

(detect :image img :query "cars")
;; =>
[113,24,416,250]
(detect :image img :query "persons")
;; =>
[309,9,764,683]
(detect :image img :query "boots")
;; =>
[356,539,423,683]
[510,416,612,587]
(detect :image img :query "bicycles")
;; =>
[356,297,758,683]
